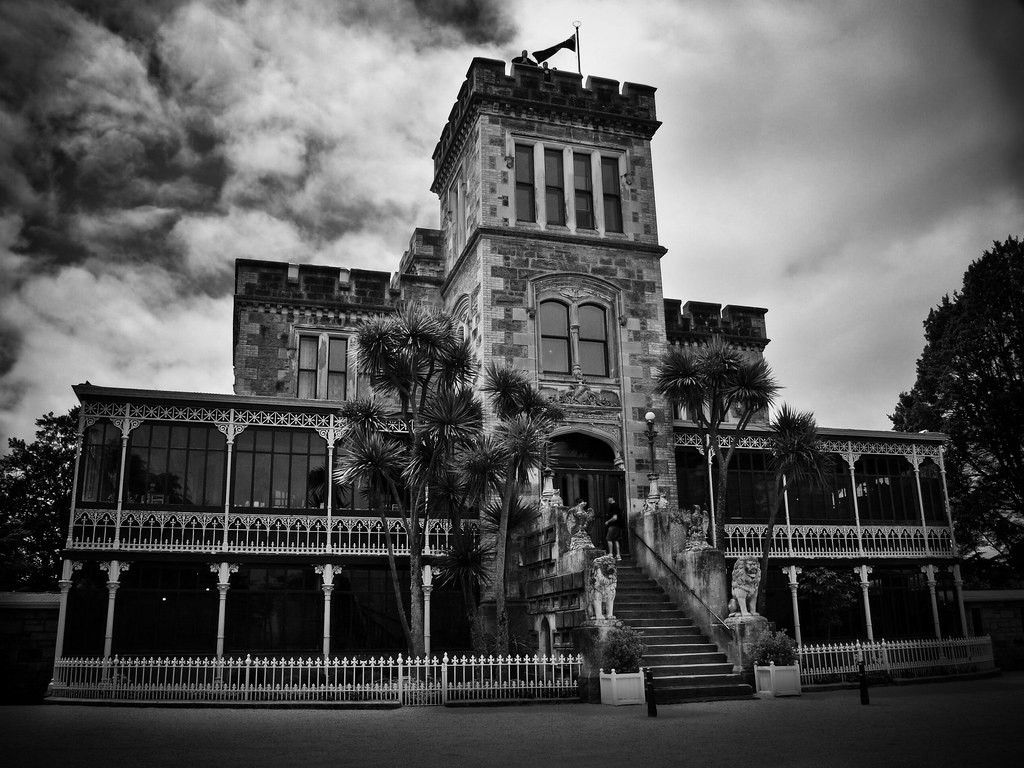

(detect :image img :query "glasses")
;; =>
[604,496,612,499]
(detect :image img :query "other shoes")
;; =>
[614,556,622,562]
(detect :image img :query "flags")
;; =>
[532,33,576,64]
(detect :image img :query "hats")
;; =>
[605,492,614,497]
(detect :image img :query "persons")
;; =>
[539,62,558,82]
[605,494,622,561]
[511,50,538,66]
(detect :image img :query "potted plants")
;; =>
[599,626,648,706]
[751,628,802,697]
